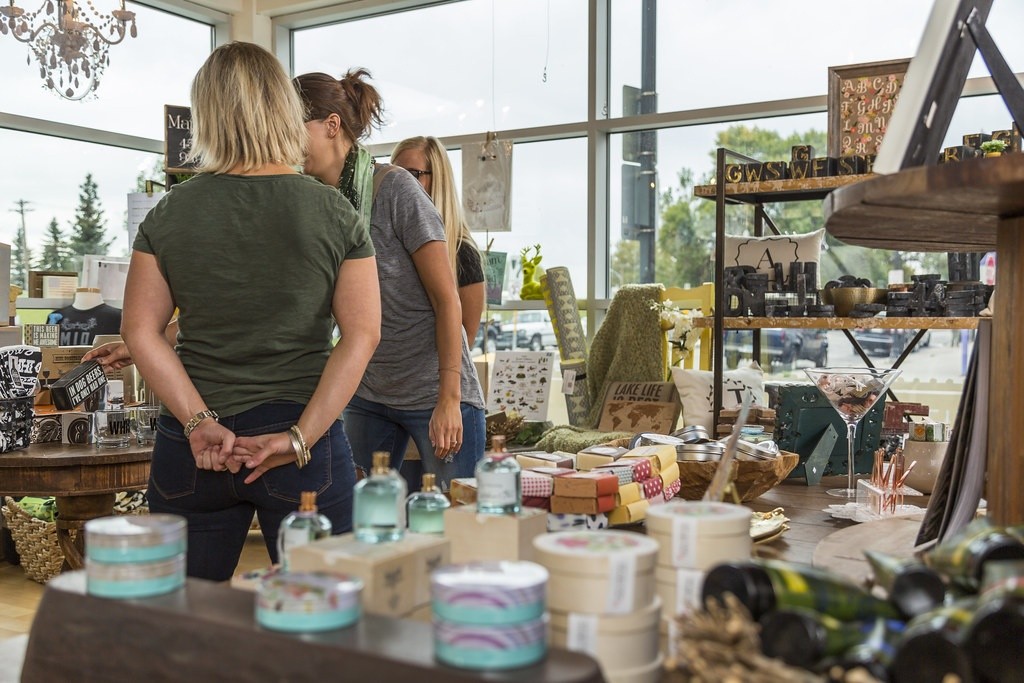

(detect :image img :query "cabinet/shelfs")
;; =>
[694,148,993,443]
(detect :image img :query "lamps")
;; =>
[0,0,138,102]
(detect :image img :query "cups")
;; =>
[99,262,130,309]
[902,437,951,493]
[94,380,160,448]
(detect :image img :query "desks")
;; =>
[16,483,932,683]
[810,147,1024,588]
[0,428,158,575]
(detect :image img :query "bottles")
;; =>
[406,473,451,533]
[10,358,22,386]
[475,436,522,514]
[278,492,332,571]
[352,452,408,541]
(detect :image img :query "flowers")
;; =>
[640,295,705,383]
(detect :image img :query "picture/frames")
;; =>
[828,57,915,176]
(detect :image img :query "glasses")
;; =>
[407,168,433,179]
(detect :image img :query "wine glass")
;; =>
[42,367,50,387]
[803,367,902,497]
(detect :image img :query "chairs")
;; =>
[621,283,716,384]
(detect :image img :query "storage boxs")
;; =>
[290,502,550,626]
[0,394,37,455]
[49,360,125,445]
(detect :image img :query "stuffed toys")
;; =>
[520,244,545,300]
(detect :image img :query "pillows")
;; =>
[670,360,765,438]
[725,228,827,292]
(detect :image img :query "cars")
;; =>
[854,328,931,358]
[724,328,829,368]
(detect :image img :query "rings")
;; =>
[222,468,227,471]
[451,441,457,443]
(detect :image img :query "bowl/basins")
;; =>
[818,287,889,317]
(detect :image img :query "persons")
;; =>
[81,71,486,494]
[391,136,485,350]
[46,287,122,345]
[120,43,382,579]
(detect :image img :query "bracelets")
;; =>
[438,368,461,374]
[287,425,311,469]
[184,410,218,438]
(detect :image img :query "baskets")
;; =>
[0,495,140,586]
